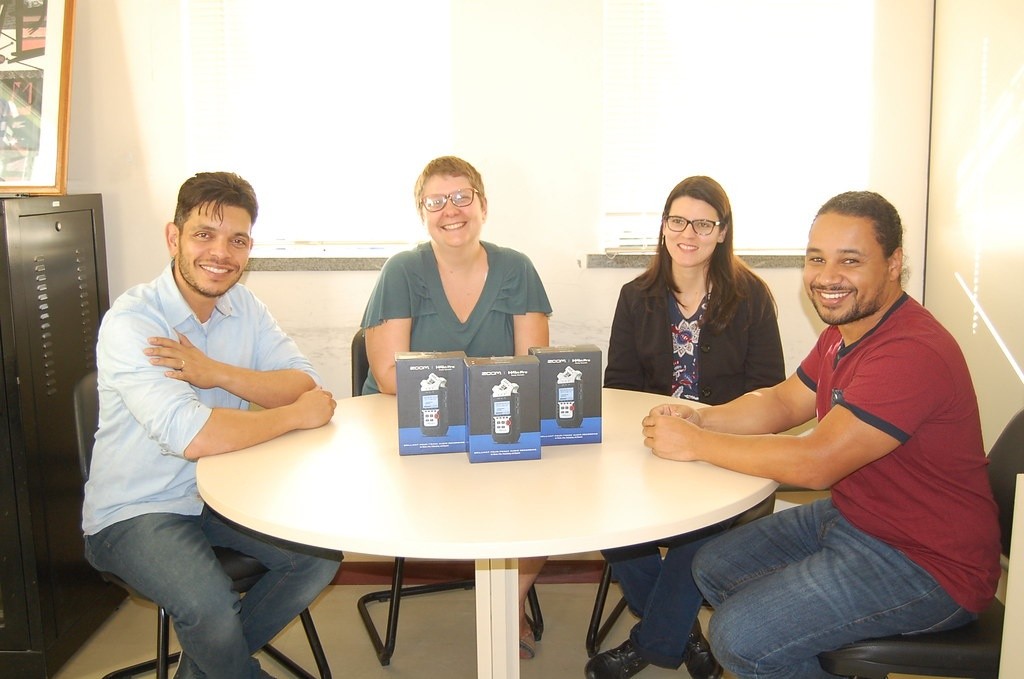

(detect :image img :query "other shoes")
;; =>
[519,607,535,658]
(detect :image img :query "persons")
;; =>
[642,190,1001,679]
[586,176,786,679]
[361,155,553,660]
[81,173,345,679]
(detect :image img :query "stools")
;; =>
[585,490,787,656]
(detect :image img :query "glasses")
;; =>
[666,215,721,236]
[420,188,478,212]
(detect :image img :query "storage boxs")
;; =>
[462,356,542,463]
[395,350,469,455]
[529,347,604,445]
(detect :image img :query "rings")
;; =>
[181,360,184,370]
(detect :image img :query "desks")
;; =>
[194,389,778,678]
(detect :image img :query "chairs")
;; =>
[352,328,545,666]
[820,413,1024,679]
[75,366,332,679]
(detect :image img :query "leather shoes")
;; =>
[585,639,650,679]
[683,617,723,679]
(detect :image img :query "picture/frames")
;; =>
[1,0,78,196]
